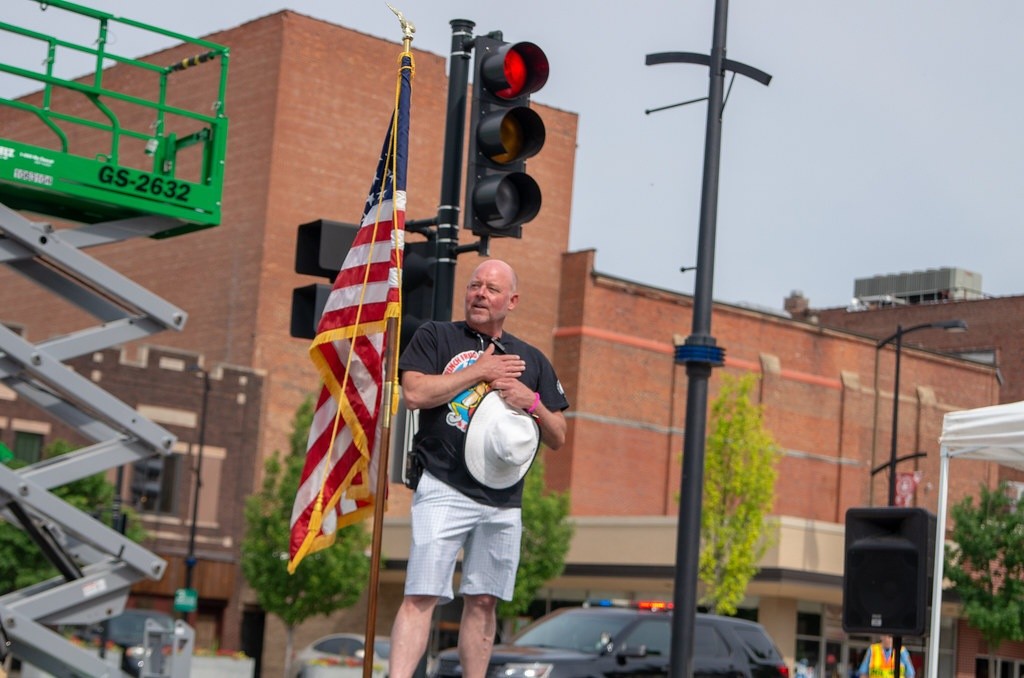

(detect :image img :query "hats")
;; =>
[464,390,540,490]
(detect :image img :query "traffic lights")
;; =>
[839,505,935,642]
[463,34,552,241]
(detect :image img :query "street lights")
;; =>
[871,318,971,504]
[175,365,217,622]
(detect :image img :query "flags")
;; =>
[287,51,416,577]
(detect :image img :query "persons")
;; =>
[387,259,570,678]
[858,633,915,678]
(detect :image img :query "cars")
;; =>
[292,632,435,678]
[85,606,183,675]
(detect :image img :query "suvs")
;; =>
[433,596,793,677]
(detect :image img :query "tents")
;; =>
[928,398,1024,678]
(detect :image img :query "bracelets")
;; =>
[528,392,540,413]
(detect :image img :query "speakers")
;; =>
[843,507,935,638]
